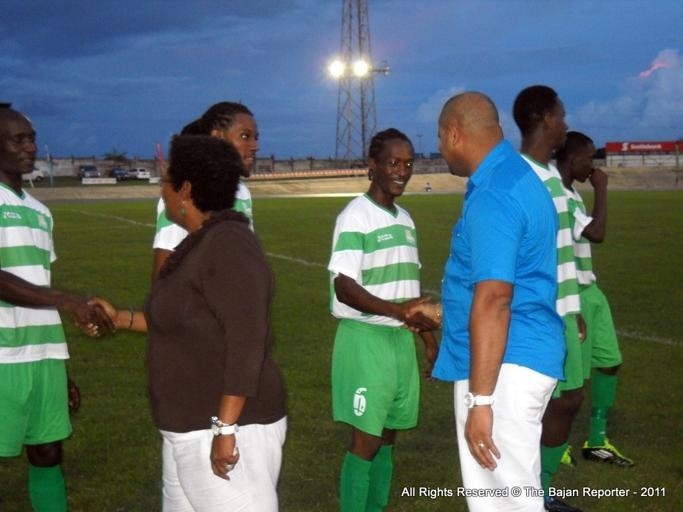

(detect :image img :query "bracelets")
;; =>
[435,303,443,323]
[123,305,137,335]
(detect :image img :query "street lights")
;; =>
[325,56,372,169]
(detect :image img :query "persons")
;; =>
[509,83,589,512]
[151,100,259,292]
[549,129,636,470]
[0,105,117,510]
[323,126,444,510]
[396,90,569,511]
[74,131,288,512]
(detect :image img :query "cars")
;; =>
[127,168,150,180]
[20,168,43,182]
[110,169,126,181]
[78,165,99,179]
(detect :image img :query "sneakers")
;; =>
[559,444,578,470]
[581,438,635,469]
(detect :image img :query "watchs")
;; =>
[203,417,241,439]
[460,390,497,411]
